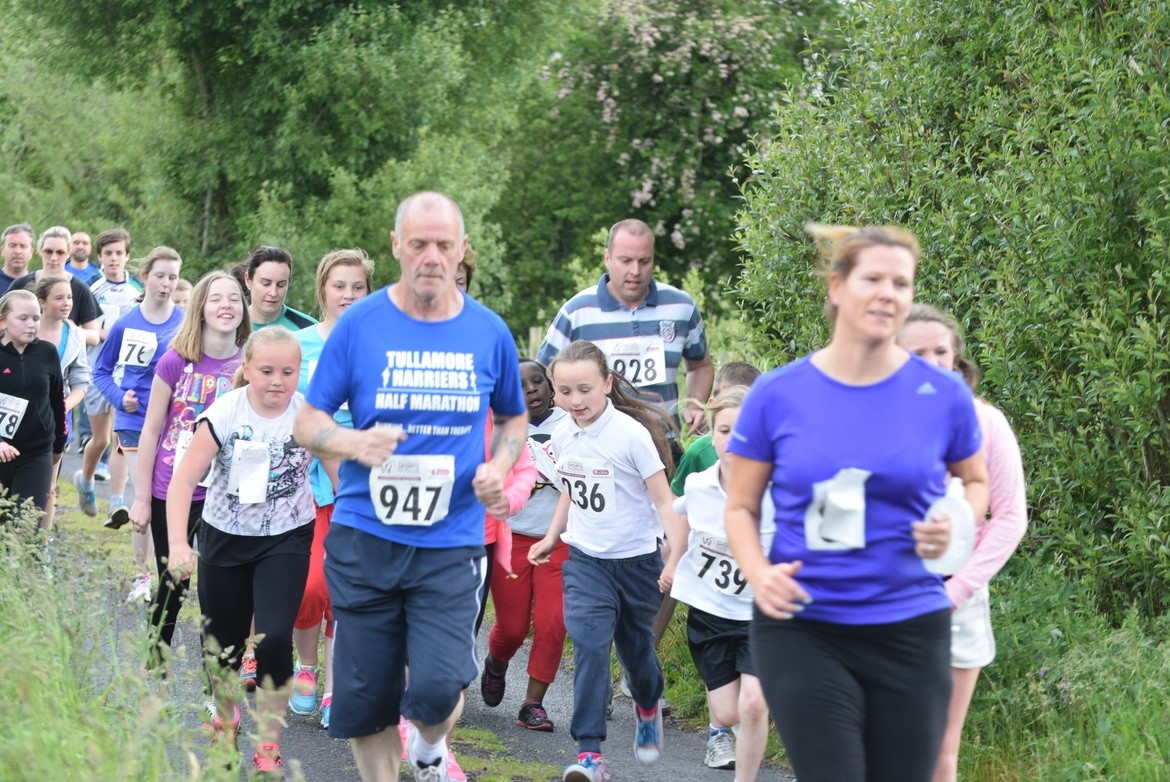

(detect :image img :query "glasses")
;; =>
[41,248,65,257]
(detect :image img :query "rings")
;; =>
[926,543,936,555]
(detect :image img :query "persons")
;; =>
[0,220,778,782]
[894,304,1028,782]
[293,190,528,782]
[724,224,992,782]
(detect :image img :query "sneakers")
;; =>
[480,653,509,706]
[73,470,98,517]
[514,701,555,733]
[288,659,319,716]
[320,696,333,728]
[562,753,609,782]
[445,751,467,782]
[406,733,448,782]
[398,715,415,762]
[619,675,671,717]
[210,705,241,771]
[633,699,664,765]
[704,734,736,769]
[240,653,257,680]
[127,575,151,602]
[251,743,283,782]
[103,504,130,530]
